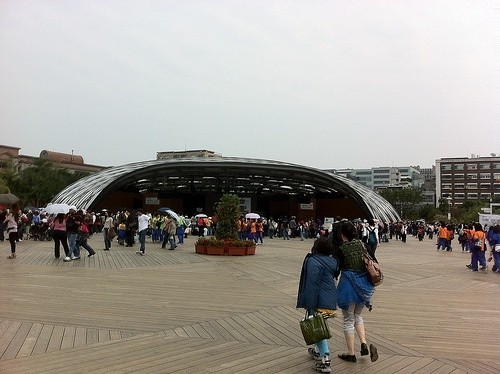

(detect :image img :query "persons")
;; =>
[297,237,339,372]
[336,221,373,363]
[237,216,341,245]
[0,205,101,262]
[355,219,473,253]
[465,223,500,273]
[101,209,217,256]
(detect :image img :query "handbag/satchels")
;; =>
[299,308,332,346]
[360,239,384,288]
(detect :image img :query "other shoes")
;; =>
[368,344,379,363]
[337,353,357,362]
[71,256,80,260]
[88,252,96,257]
[62,257,71,261]
[360,343,369,356]
[466,263,500,273]
[9,253,15,258]
[315,360,331,373]
[308,347,321,361]
[136,251,142,254]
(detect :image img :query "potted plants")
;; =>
[194,192,257,255]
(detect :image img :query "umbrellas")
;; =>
[45,204,69,214]
[197,214,207,218]
[246,213,260,219]
[165,210,179,220]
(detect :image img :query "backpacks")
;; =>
[366,226,378,246]
